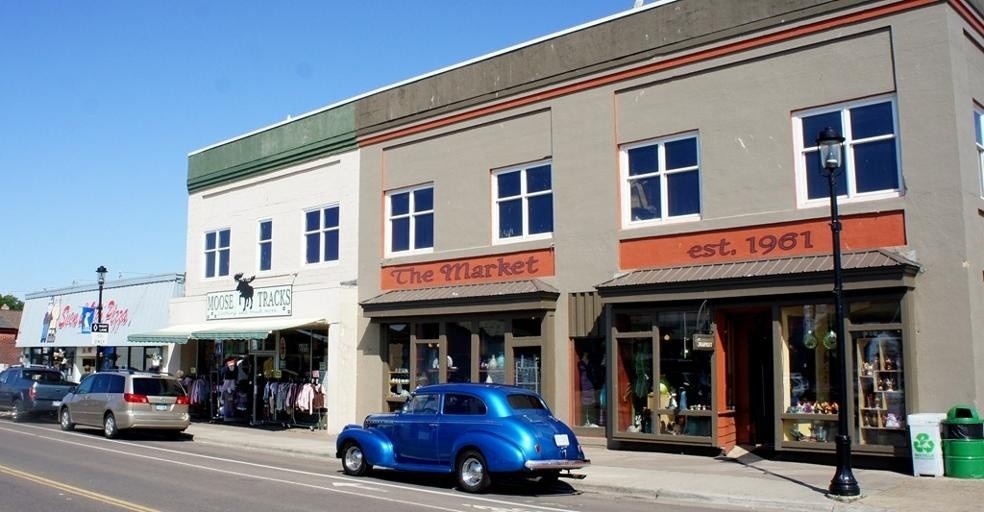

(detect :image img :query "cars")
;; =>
[329,376,592,501]
[790,369,810,402]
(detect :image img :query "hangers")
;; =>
[266,378,324,386]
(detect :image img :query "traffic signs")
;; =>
[91,323,112,347]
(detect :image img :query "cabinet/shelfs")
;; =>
[386,368,411,404]
[855,335,909,447]
[657,408,712,443]
[781,412,840,449]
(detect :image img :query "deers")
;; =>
[235,274,255,311]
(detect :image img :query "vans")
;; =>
[57,368,193,438]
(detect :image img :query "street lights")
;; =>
[94,264,112,373]
[812,124,871,499]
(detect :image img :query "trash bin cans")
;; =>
[907,403,984,480]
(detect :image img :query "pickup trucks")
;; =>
[1,364,80,423]
